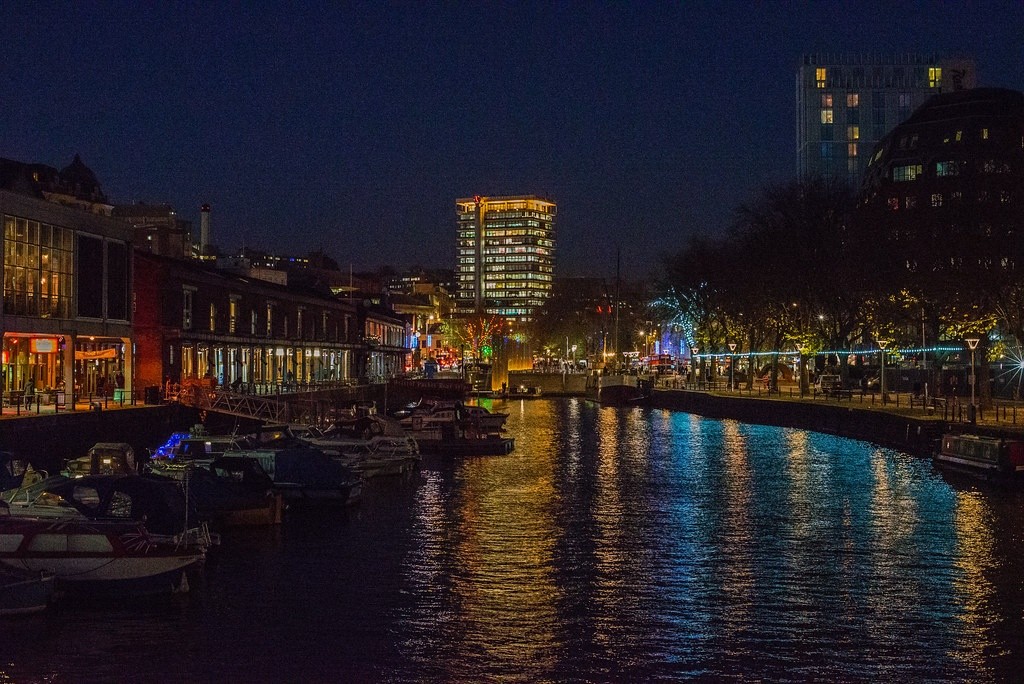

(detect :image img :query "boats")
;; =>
[935,431,1024,472]
[377,396,514,460]
[27,441,290,540]
[151,427,382,511]
[244,414,435,480]
[0,458,219,580]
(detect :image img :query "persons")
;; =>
[913,380,921,398]
[808,370,816,382]
[861,374,870,398]
[115,370,125,389]
[532,359,762,392]
[24,377,34,411]
[286,369,293,393]
[95,373,106,398]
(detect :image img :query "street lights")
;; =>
[876,340,890,408]
[795,342,805,399]
[965,338,980,423]
[729,344,737,391]
[545,344,699,390]
